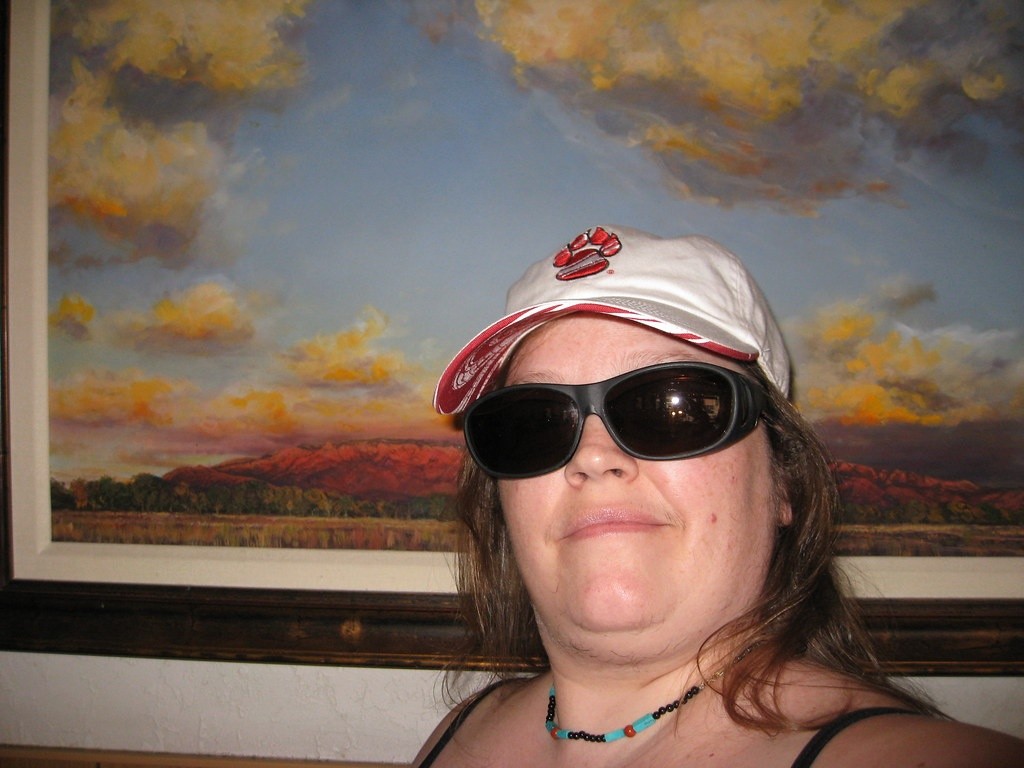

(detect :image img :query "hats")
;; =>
[434,224,790,415]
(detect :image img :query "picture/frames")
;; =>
[1,0,1024,680]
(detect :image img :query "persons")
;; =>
[412,228,1024,768]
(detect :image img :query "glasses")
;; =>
[452,361,780,480]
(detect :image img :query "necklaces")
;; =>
[546,639,767,744]
